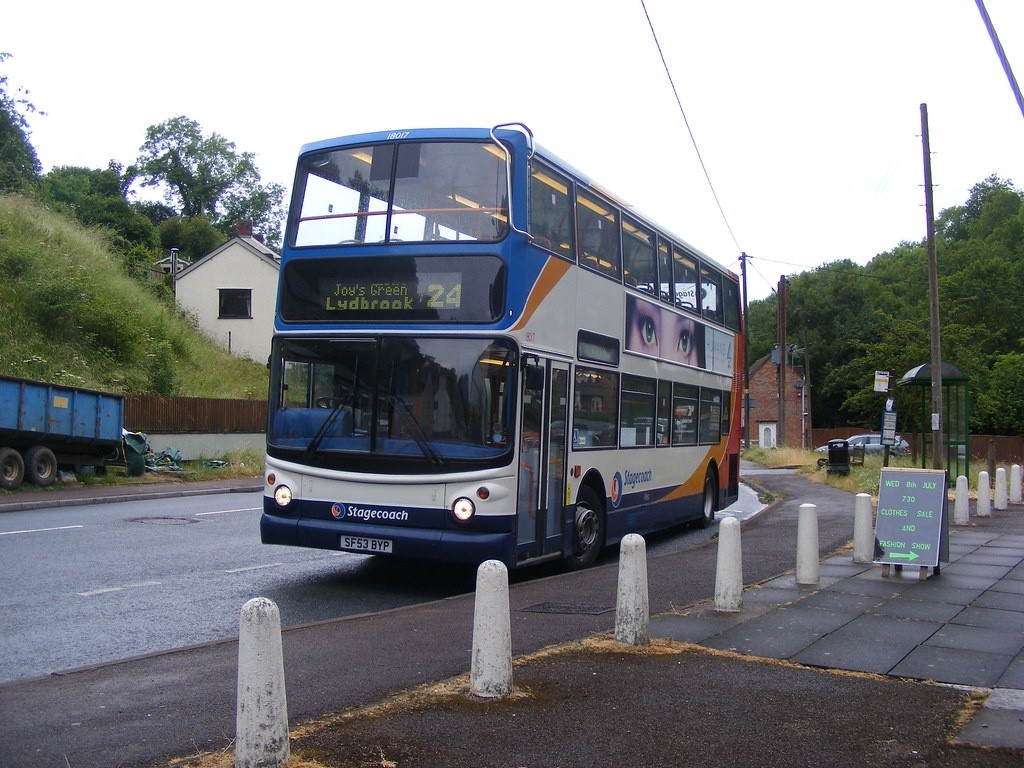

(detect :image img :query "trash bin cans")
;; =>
[826,439,851,476]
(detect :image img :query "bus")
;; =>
[259,130,744,571]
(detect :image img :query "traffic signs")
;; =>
[871,469,945,566]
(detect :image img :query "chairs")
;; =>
[338,233,717,319]
[333,381,373,414]
[850,443,865,468]
[551,402,672,448]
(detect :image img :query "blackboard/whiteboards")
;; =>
[871,466,951,567]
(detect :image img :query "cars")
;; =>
[815,434,912,458]
[623,415,668,431]
[550,419,564,442]
